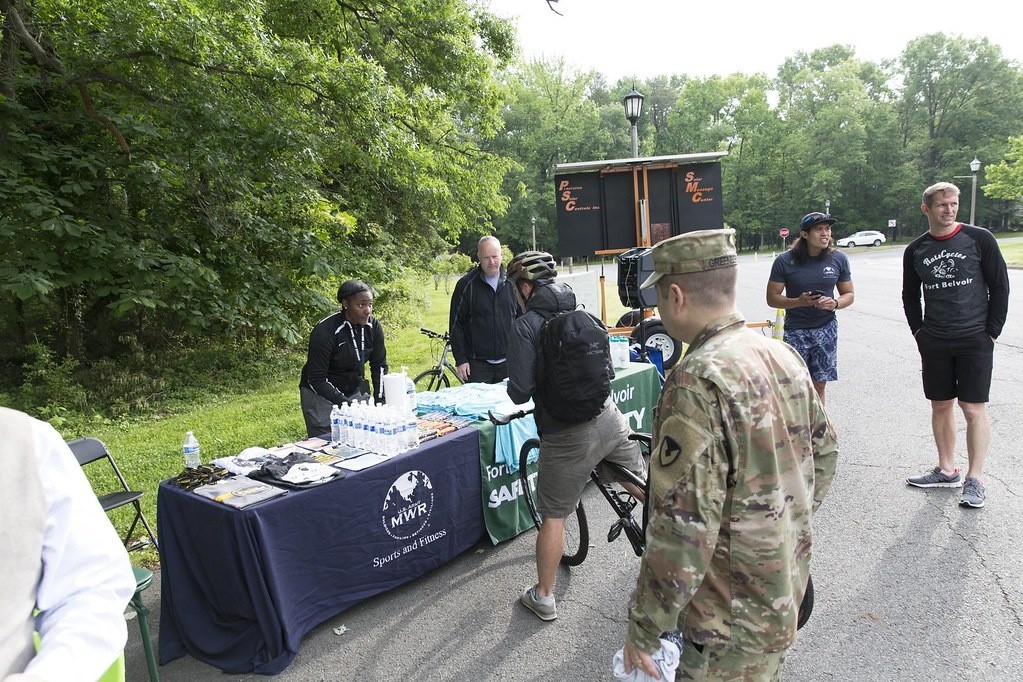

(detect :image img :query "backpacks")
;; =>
[532,304,611,424]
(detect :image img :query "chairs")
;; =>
[65,436,162,554]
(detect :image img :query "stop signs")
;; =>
[780,228,789,238]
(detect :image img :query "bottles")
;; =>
[609,334,630,369]
[184,431,202,470]
[659,630,684,654]
[330,398,420,456]
[401,367,418,414]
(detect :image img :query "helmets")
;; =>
[506,251,557,282]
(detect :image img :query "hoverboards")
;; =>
[616,310,683,370]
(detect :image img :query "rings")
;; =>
[633,660,641,664]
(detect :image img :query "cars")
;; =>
[837,230,886,248]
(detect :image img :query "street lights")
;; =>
[969,155,981,226]
[622,83,644,158]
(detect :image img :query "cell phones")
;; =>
[810,290,826,300]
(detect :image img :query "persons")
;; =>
[505,252,646,622]
[623,229,839,682]
[901,182,1010,509]
[449,235,525,383]
[299,279,389,438]
[766,212,855,406]
[0,405,137,682]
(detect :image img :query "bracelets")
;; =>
[834,299,838,308]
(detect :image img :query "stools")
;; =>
[127,566,160,682]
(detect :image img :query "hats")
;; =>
[640,228,737,290]
[800,212,837,231]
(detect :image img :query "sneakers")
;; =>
[959,477,986,508]
[906,466,962,488]
[521,582,557,621]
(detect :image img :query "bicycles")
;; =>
[487,408,814,631]
[412,328,466,391]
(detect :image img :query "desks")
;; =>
[156,361,664,674]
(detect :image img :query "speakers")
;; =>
[618,246,658,309]
